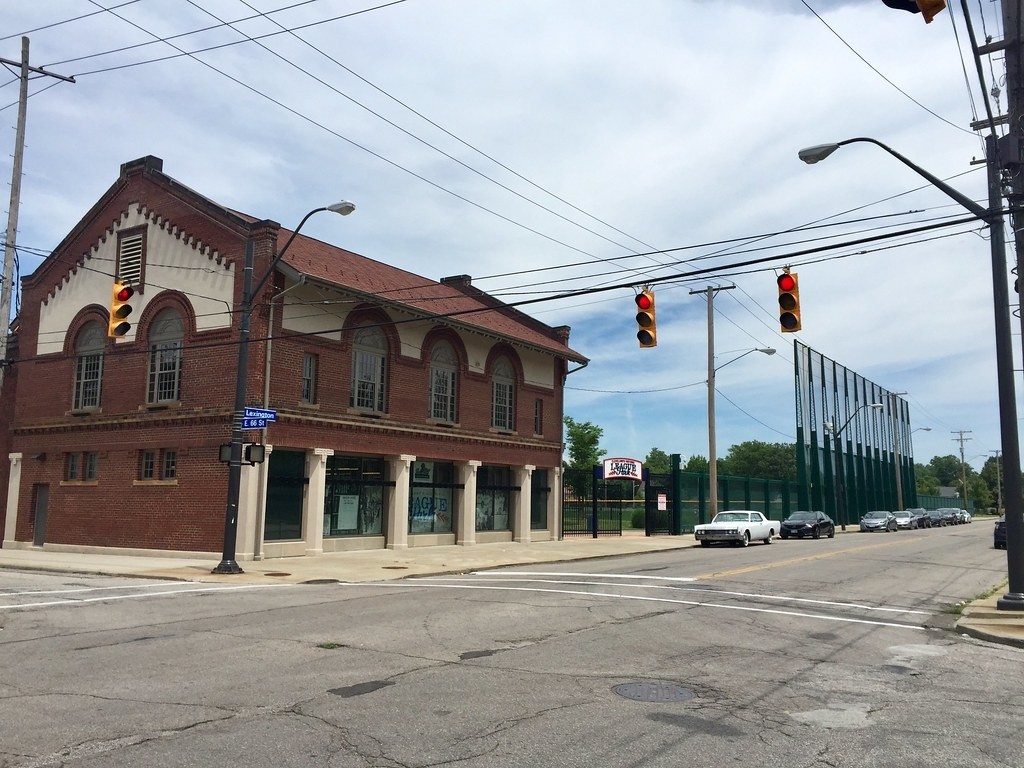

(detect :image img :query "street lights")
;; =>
[830,402,885,531]
[797,131,1024,612]
[961,454,989,512]
[209,197,359,574]
[893,427,932,511]
[706,345,777,522]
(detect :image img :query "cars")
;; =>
[994,513,1007,550]
[694,509,781,547]
[892,511,919,530]
[906,506,973,529]
[859,510,898,532]
[781,510,835,540]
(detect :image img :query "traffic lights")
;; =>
[246,442,266,464]
[218,444,232,462]
[776,271,802,332]
[635,291,657,349]
[106,283,134,339]
